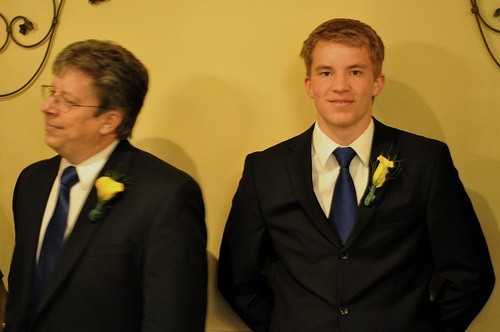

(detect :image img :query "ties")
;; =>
[28,166,80,312]
[328,147,359,248]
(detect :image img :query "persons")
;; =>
[215,18,496,332]
[0,38,208,332]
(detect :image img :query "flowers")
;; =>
[362,146,405,203]
[87,172,125,222]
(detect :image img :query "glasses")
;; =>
[41,85,111,113]
